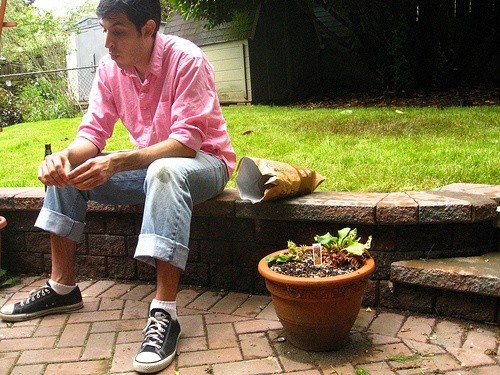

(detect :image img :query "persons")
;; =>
[1,0,237,372]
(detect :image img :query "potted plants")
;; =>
[258,228,376,351]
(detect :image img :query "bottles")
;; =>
[44,143,52,192]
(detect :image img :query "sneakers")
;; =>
[133,308,182,373]
[0,280,83,321]
